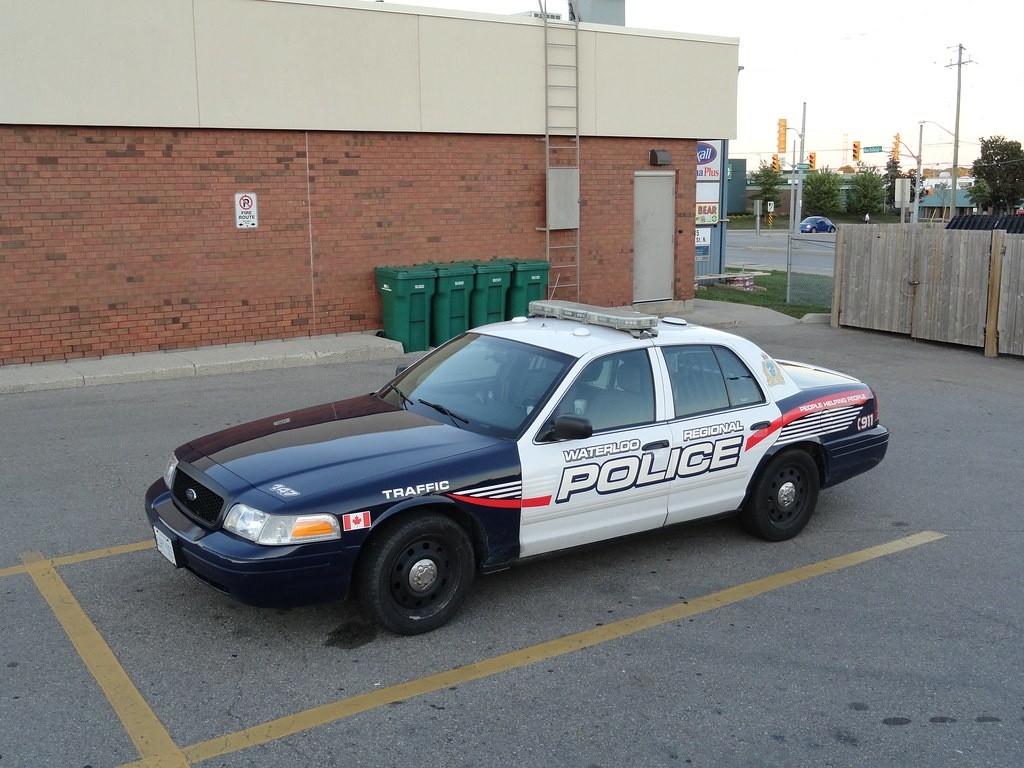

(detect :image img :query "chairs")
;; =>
[534,361,602,417]
[585,364,645,430]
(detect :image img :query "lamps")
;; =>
[650,149,672,166]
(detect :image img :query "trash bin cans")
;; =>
[491,257,552,320]
[452,258,513,329]
[375,265,438,353]
[414,261,476,347]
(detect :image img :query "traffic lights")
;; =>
[852,141,860,161]
[928,189,933,195]
[810,153,816,170]
[772,154,780,172]
[891,133,900,161]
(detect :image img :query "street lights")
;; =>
[894,125,922,223]
[884,137,921,225]
[919,121,959,222]
[786,128,805,234]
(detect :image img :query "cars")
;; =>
[800,216,836,233]
[145,299,891,635]
